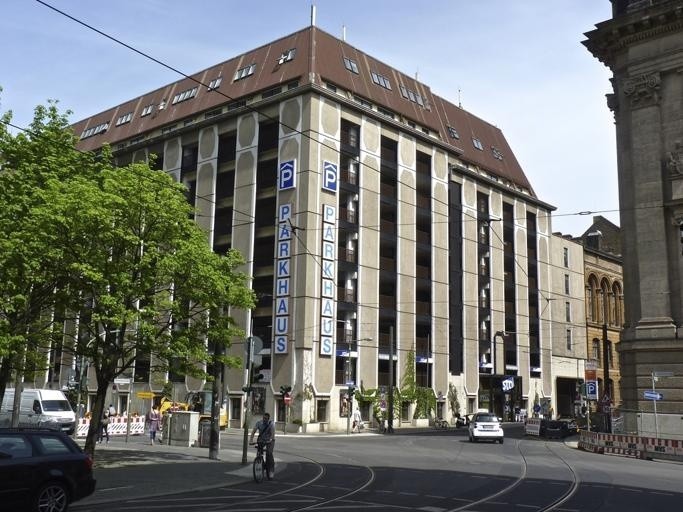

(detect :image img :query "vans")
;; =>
[0,388,77,435]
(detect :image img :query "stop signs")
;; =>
[284,392,293,404]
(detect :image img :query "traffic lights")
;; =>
[349,387,355,396]
[279,386,292,394]
[251,362,264,383]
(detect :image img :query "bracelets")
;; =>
[269,439,271,440]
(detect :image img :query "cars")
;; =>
[0,428,97,511]
[542,421,570,439]
[468,412,504,443]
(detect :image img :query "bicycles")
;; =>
[252,442,274,483]
[434,417,450,432]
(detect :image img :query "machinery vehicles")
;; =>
[137,392,228,430]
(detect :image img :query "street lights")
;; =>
[494,331,509,374]
[347,338,374,435]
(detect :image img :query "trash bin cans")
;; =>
[199,415,213,448]
[599,413,610,433]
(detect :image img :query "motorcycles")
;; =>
[455,414,471,428]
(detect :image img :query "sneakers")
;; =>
[268,469,274,478]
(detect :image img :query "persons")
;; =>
[581,403,587,413]
[77,402,86,418]
[249,412,276,479]
[157,411,167,443]
[145,405,160,446]
[109,404,116,418]
[548,404,555,421]
[101,409,110,444]
[350,407,361,433]
